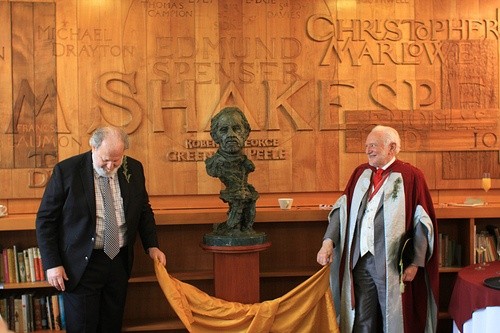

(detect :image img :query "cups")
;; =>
[278,198,293,210]
[0,204,7,216]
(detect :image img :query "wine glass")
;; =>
[482,173,491,207]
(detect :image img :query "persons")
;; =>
[317,124,438,333]
[209,107,250,156]
[36,126,166,333]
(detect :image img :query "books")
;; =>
[0,294,65,333]
[473,225,500,265]
[0,246,46,284]
[439,233,461,267]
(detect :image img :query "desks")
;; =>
[449,260,500,333]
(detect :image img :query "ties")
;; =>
[374,168,383,189]
[100,177,120,260]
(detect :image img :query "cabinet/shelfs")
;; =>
[0,217,500,333]
